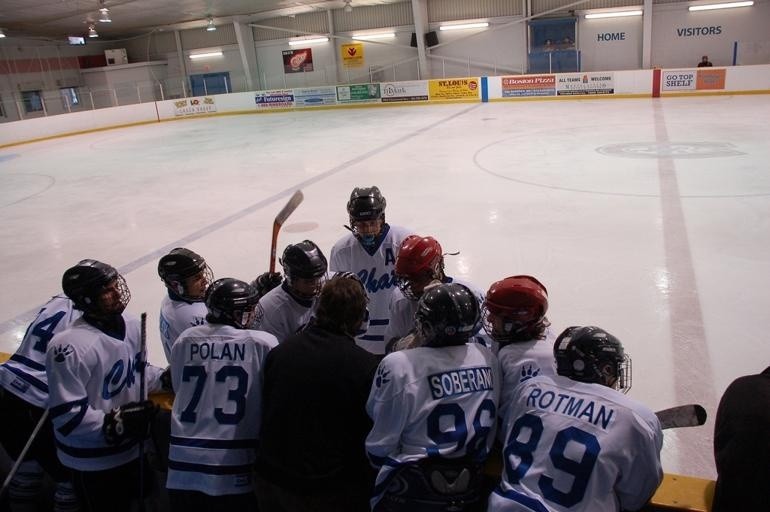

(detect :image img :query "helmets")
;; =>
[554,325,632,394]
[279,240,327,278]
[62,259,131,314]
[394,235,551,344]
[158,248,260,329]
[347,186,385,220]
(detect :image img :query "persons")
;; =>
[245,239,331,345]
[43,256,173,511]
[1,291,87,510]
[329,187,416,365]
[484,325,665,509]
[158,243,284,370]
[383,234,495,354]
[696,53,714,68]
[167,276,281,512]
[480,270,560,409]
[711,365,770,512]
[248,271,376,509]
[365,277,503,510]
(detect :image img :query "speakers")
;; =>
[425,31,438,47]
[411,33,417,47]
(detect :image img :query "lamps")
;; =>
[205,16,216,31]
[98,4,112,22]
[344,0,352,12]
[87,21,99,37]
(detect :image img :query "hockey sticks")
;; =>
[270,190,303,273]
[655,404,706,430]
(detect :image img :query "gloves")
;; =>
[257,271,282,295]
[104,400,155,445]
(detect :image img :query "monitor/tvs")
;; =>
[67,36,86,46]
